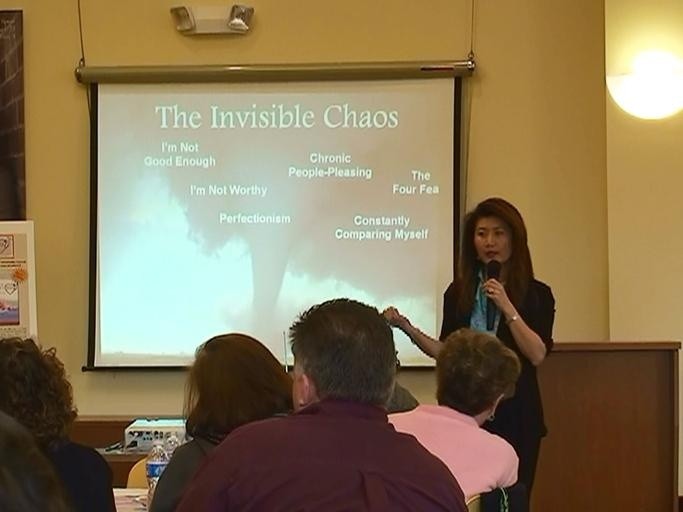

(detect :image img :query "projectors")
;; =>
[123,418,188,452]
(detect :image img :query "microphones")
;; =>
[485,259,502,331]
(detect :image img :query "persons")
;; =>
[0,413,71,511]
[148,332,293,511]
[1,337,117,510]
[387,382,421,413]
[387,326,522,507]
[171,298,470,511]
[383,198,555,511]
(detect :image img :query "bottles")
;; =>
[146,440,167,511]
[163,436,181,459]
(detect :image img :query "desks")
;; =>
[93,446,174,488]
[113,488,151,511]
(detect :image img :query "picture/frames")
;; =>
[1,221,35,344]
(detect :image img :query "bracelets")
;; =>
[505,311,518,324]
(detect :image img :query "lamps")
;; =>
[605,51,683,120]
